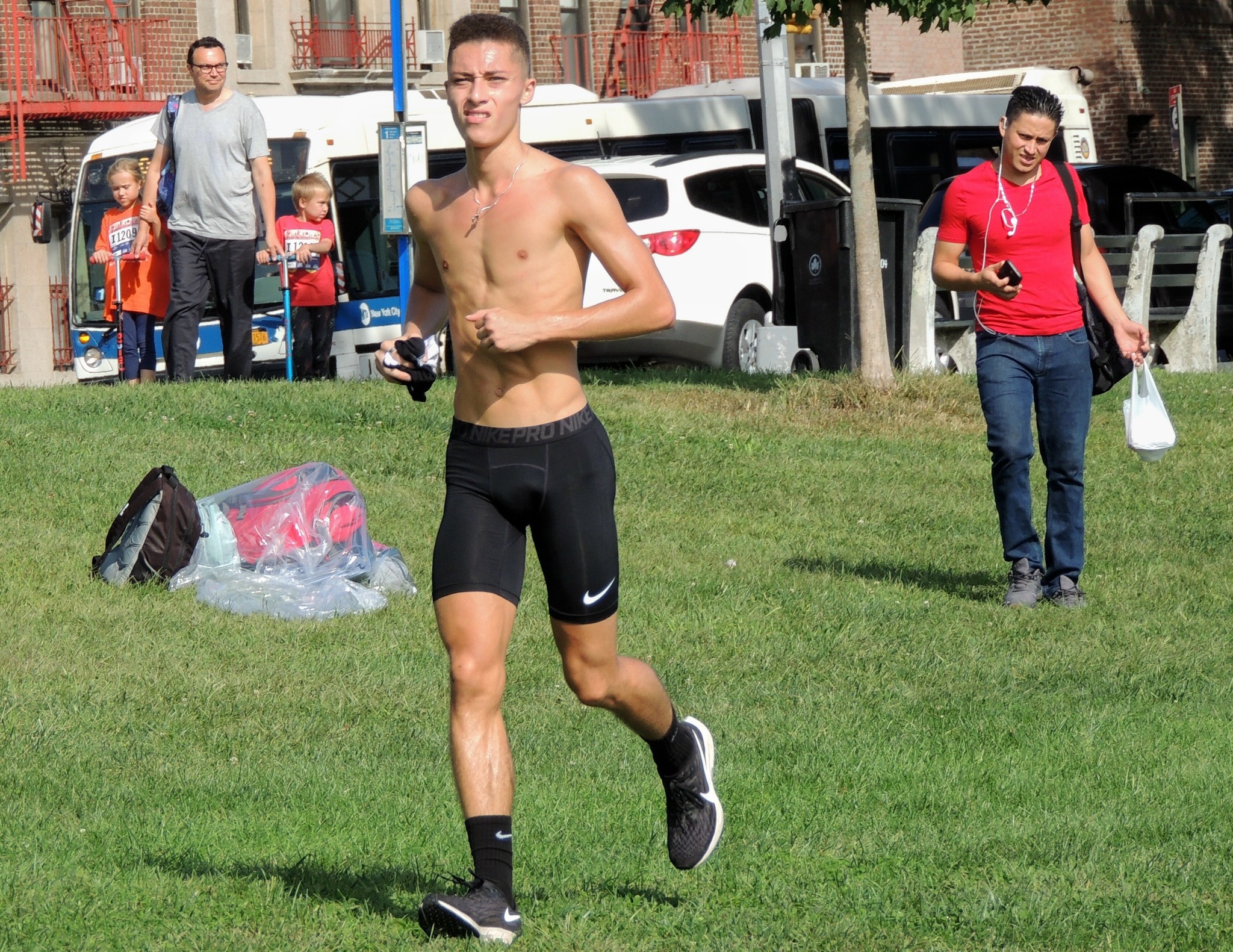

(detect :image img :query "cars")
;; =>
[917,159,1233,364]
[559,149,851,375]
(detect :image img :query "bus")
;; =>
[27,64,1101,384]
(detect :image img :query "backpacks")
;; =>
[189,497,239,579]
[212,461,376,588]
[90,466,207,593]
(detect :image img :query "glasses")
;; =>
[192,62,229,74]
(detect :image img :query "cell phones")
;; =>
[996,259,1022,286]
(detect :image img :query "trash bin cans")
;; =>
[772,197,923,375]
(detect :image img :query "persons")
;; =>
[93,158,174,385]
[930,86,1151,609]
[255,171,336,381]
[372,13,726,945]
[132,36,286,384]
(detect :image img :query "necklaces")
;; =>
[465,143,530,225]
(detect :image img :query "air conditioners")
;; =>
[109,56,143,85]
[796,62,830,78]
[683,61,711,84]
[236,34,253,64]
[416,29,445,64]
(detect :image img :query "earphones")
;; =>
[1003,118,1008,130]
[1008,227,1016,236]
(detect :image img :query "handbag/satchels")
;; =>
[1121,357,1175,461]
[154,94,183,214]
[1071,276,1134,395]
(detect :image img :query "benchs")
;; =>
[906,223,1233,378]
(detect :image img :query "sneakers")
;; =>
[1044,574,1086,612]
[418,866,521,947]
[1005,556,1043,608]
[661,716,724,872]
